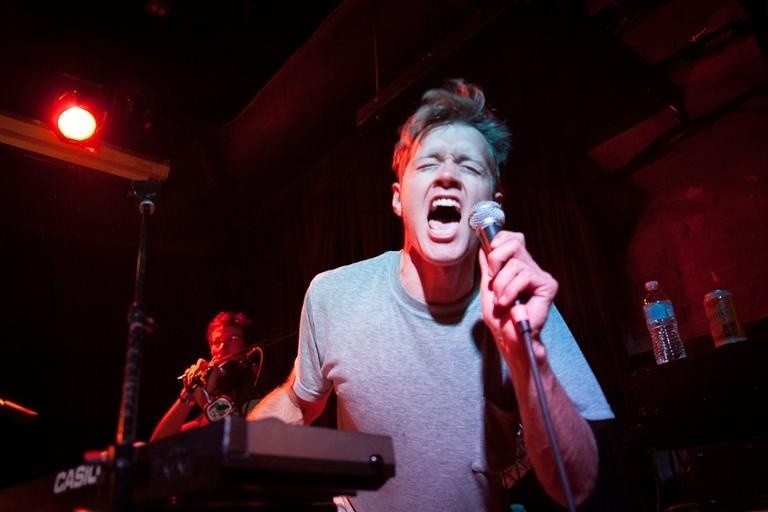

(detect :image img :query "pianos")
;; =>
[0,414,396,511]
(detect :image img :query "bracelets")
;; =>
[178,394,190,406]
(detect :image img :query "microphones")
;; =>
[468,199,531,334]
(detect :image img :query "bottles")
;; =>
[642,281,687,364]
[700,270,745,349]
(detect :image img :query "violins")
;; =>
[176,346,264,417]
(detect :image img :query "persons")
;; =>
[149,310,265,443]
[244,78,615,512]
